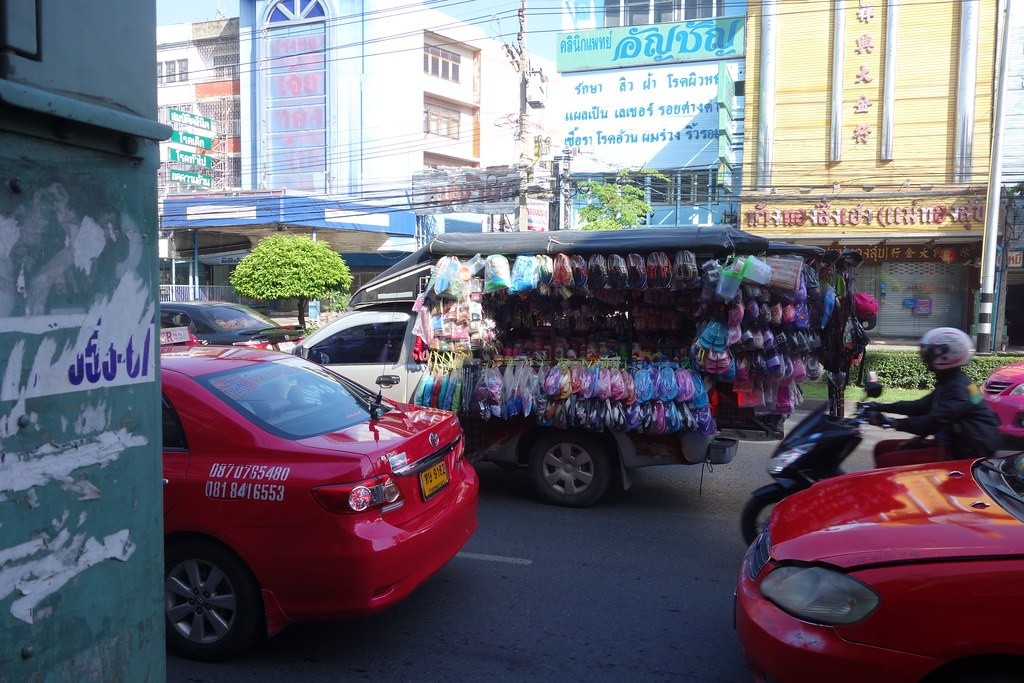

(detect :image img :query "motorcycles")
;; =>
[740,369,1024,550]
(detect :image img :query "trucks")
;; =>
[280,225,792,509]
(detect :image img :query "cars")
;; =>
[982,361,1024,438]
[731,448,1024,683]
[160,301,306,358]
[162,343,479,661]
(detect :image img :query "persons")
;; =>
[867,327,1004,461]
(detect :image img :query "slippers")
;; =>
[413,251,824,435]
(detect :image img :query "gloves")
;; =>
[868,411,890,427]
[856,401,882,411]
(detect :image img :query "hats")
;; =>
[853,293,878,331]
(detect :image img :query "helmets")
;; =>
[918,326,976,371]
